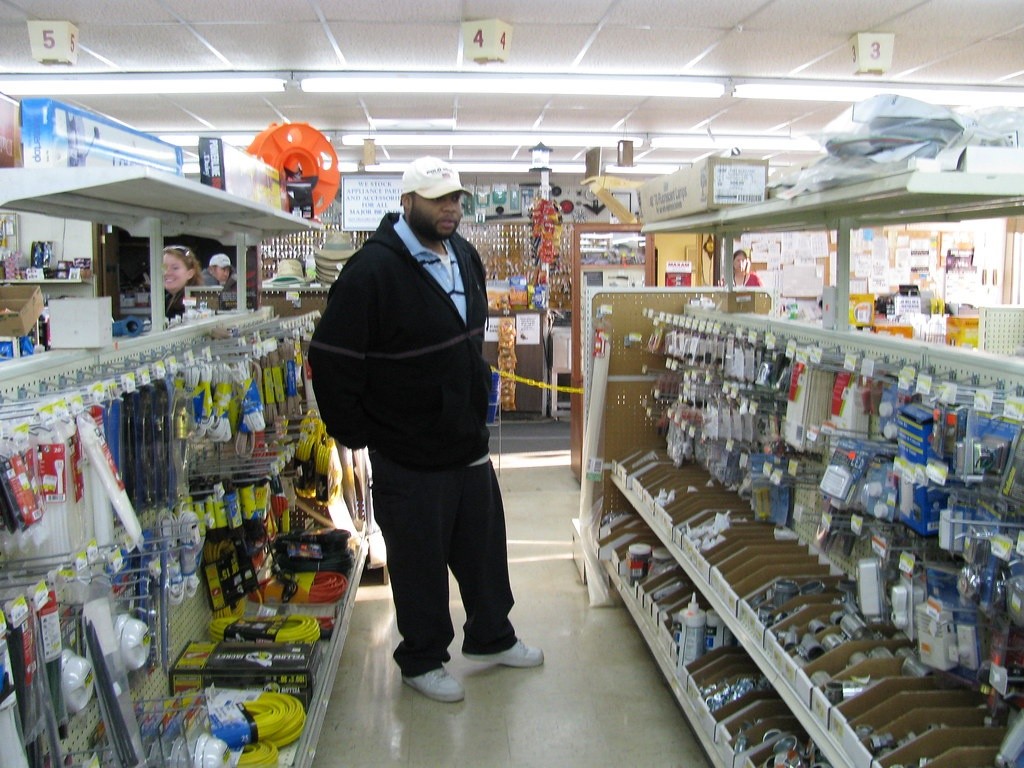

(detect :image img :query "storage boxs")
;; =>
[17,95,185,178]
[57,260,73,270]
[840,690,1007,768]
[47,295,113,349]
[945,316,979,349]
[167,640,324,714]
[717,702,793,758]
[723,718,805,768]
[849,293,874,327]
[69,267,92,280]
[0,286,43,337]
[940,145,1024,174]
[198,137,289,214]
[828,677,975,745]
[892,402,943,487]
[56,270,69,279]
[702,690,782,744]
[74,257,90,270]
[897,478,948,536]
[636,156,770,225]
[871,726,1008,768]
[593,448,920,710]
[872,324,913,339]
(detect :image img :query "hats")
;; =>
[313,233,358,285]
[209,253,230,268]
[267,259,315,284]
[401,155,473,199]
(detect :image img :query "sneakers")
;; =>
[401,665,464,702]
[462,637,544,667]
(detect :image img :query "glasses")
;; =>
[167,246,190,260]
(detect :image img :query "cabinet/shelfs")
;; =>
[0,165,387,768]
[592,305,1024,768]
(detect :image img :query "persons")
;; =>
[202,252,234,290]
[160,246,206,321]
[732,251,763,289]
[308,155,545,702]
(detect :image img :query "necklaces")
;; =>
[733,272,746,286]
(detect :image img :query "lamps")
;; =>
[617,120,636,168]
[638,133,657,158]
[0,71,289,93]
[528,121,553,172]
[143,129,336,147]
[648,132,821,152]
[728,77,1024,108]
[292,71,731,99]
[362,124,380,166]
[334,130,647,149]
